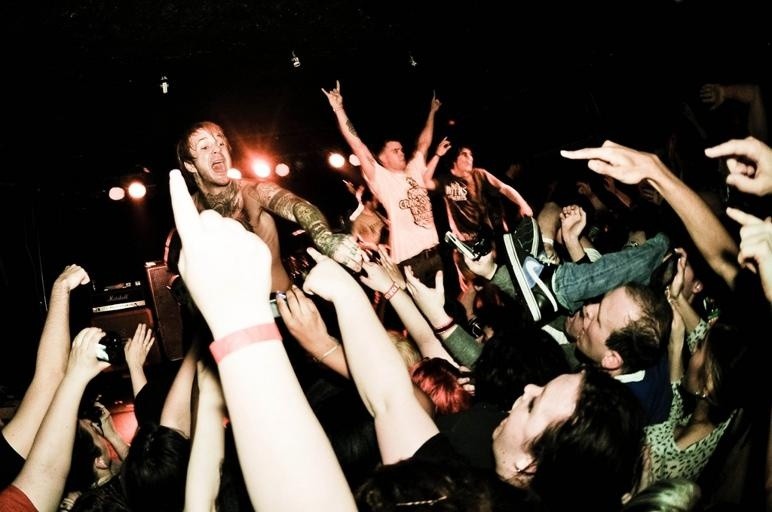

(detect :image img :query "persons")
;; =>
[1,81,770,512]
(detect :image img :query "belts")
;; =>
[400,244,439,265]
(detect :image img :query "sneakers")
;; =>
[503,216,559,323]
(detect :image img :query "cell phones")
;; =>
[270,297,291,318]
[97,341,123,366]
[446,231,478,260]
